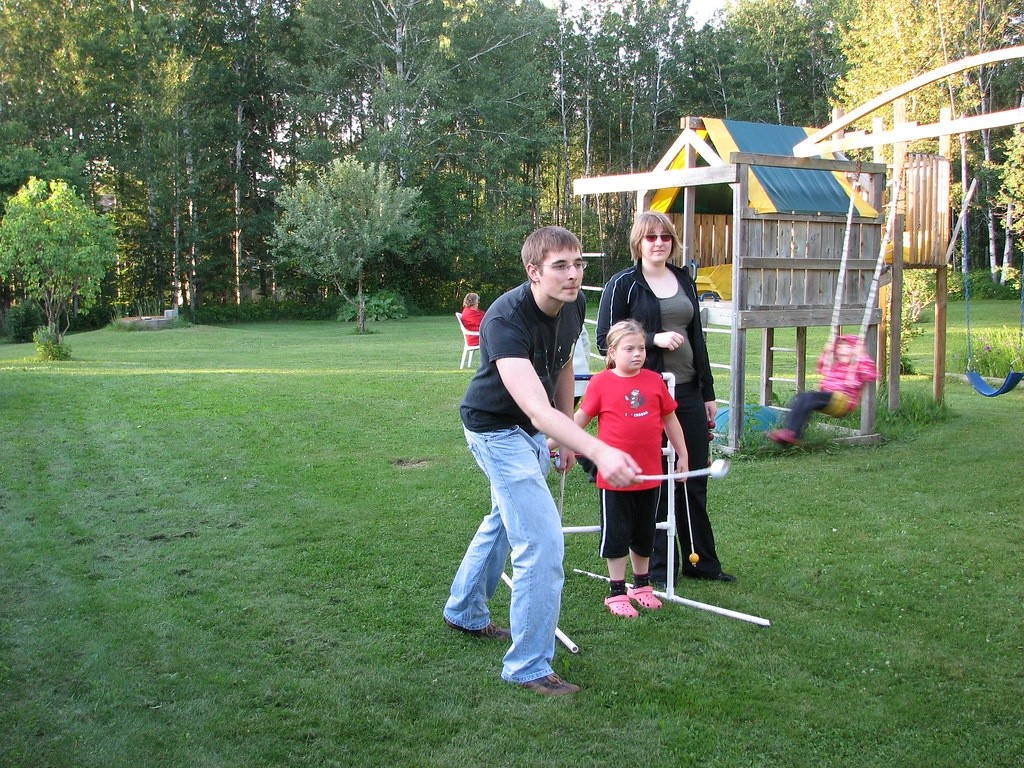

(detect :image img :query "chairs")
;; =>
[454,313,485,368]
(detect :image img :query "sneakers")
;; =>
[517,673,581,698]
[443,613,511,641]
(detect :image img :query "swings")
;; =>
[819,140,911,418]
[959,131,1024,399]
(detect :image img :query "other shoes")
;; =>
[765,428,800,448]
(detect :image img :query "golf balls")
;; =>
[557,458,567,469]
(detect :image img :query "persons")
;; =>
[571,323,598,482]
[443,225,643,695]
[766,336,877,444]
[596,212,736,592]
[462,293,486,346]
[547,319,689,618]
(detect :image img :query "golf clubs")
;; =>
[636,458,730,481]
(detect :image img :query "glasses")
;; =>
[642,233,673,242]
[538,260,589,271]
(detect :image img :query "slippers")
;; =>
[605,594,638,617]
[627,585,663,609]
